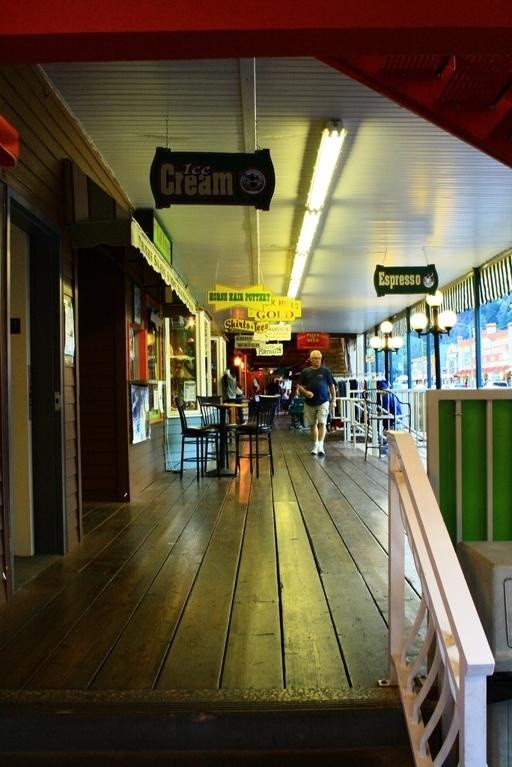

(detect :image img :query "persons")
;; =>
[297,350,336,455]
[253,368,299,416]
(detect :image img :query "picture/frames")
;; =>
[130,382,151,445]
[133,285,143,325]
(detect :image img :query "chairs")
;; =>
[174,392,282,483]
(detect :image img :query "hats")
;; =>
[307,350,322,360]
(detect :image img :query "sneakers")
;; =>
[311,447,325,456]
